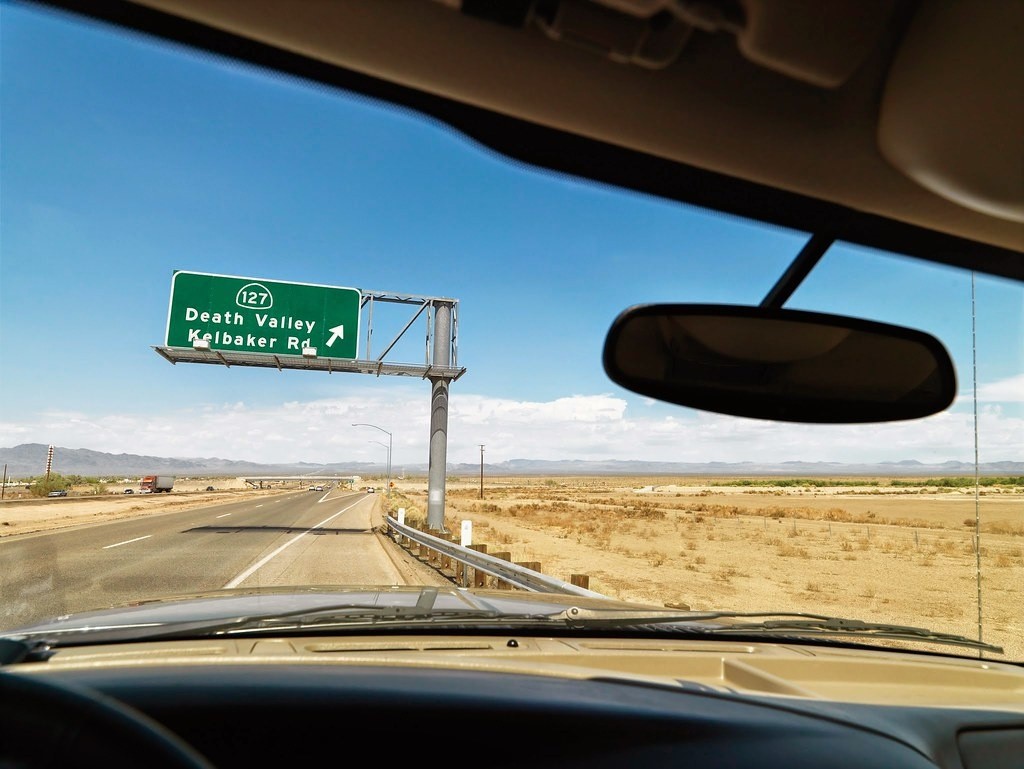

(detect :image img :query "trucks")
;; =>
[139,475,175,493]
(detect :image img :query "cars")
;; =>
[48,489,67,497]
[367,488,374,493]
[309,485,315,491]
[123,489,134,494]
[206,486,214,492]
[316,486,324,491]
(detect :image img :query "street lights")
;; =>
[366,440,389,494]
[352,423,392,499]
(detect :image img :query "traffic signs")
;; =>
[164,269,362,365]
